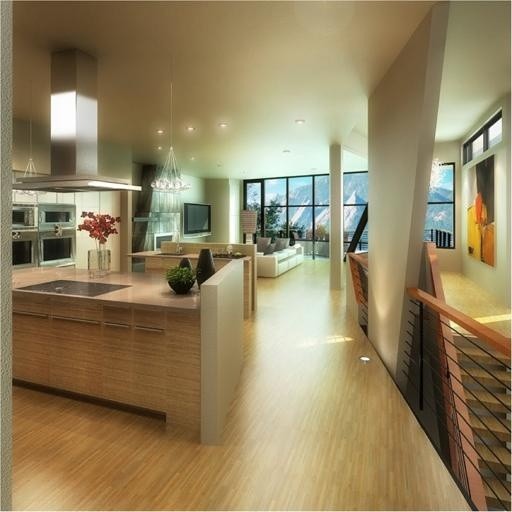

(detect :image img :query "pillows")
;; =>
[255,237,291,255]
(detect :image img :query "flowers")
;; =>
[79,211,121,271]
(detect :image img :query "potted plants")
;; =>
[164,265,195,295]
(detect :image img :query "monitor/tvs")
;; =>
[182,202,212,239]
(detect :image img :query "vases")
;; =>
[87,247,112,271]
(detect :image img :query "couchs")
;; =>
[253,240,304,278]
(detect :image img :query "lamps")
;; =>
[149,76,192,192]
